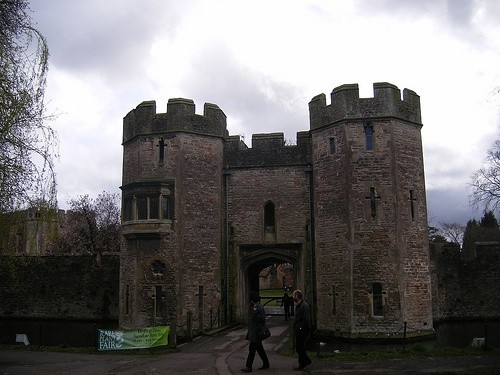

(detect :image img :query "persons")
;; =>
[279,293,291,320]
[240,291,270,373]
[292,290,313,370]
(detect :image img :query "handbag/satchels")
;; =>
[255,326,271,342]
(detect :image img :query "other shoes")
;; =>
[257,366,269,370]
[241,366,252,372]
[293,359,312,370]
